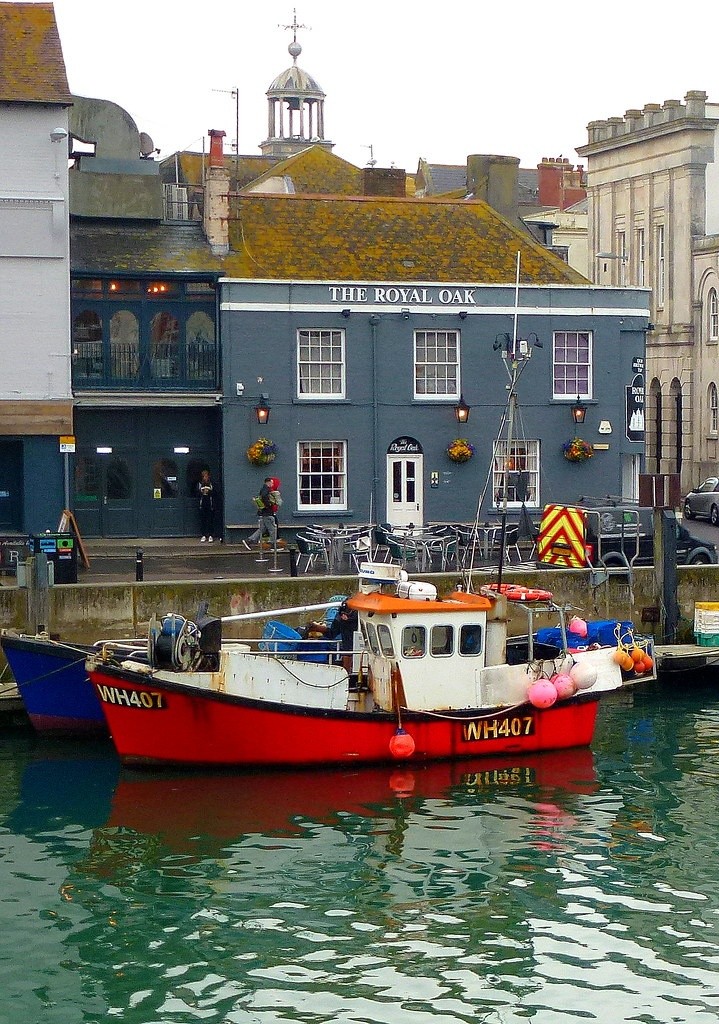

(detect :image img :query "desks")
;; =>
[322,523,360,562]
[405,534,440,573]
[464,525,503,560]
[311,534,352,570]
[394,525,431,546]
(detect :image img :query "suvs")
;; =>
[536,494,718,569]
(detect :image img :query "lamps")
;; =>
[342,309,350,317]
[570,395,588,423]
[458,312,467,320]
[403,312,410,320]
[453,393,471,423]
[642,323,655,331]
[50,127,67,142]
[254,393,271,424]
[595,252,629,261]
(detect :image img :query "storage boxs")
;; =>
[694,601,719,646]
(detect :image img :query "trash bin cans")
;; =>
[28,532,77,584]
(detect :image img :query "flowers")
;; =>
[445,438,476,464]
[246,438,279,467]
[561,437,596,463]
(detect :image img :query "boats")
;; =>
[83,580,602,764]
[1,596,656,728]
[76,743,599,875]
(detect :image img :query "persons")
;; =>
[199,470,216,543]
[242,477,284,550]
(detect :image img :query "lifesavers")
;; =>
[480,582,553,601]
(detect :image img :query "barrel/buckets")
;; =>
[536,618,634,651]
[258,621,302,652]
[297,637,328,662]
[326,596,347,650]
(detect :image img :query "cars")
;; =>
[684,476,719,527]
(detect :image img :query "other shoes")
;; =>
[242,539,252,550]
[201,535,206,542]
[270,545,284,551]
[208,536,214,543]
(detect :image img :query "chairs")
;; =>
[294,524,541,573]
